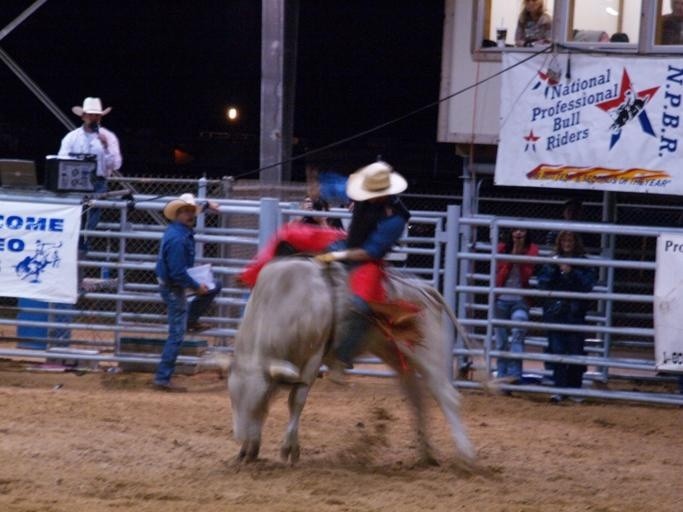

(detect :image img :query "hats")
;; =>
[70,95,114,117]
[344,160,409,201]
[162,193,205,219]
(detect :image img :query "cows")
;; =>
[201,251,496,474]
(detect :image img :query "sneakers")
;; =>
[550,392,562,406]
[328,359,349,388]
[155,381,191,394]
[188,321,219,332]
[565,396,584,402]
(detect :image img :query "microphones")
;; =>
[89,120,100,136]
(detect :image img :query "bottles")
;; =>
[495,17,507,48]
[100,260,109,279]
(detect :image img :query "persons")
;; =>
[290,130,306,167]
[55,97,124,293]
[490,226,539,396]
[515,0,553,47]
[608,33,629,42]
[300,197,344,231]
[274,161,411,384]
[545,197,594,250]
[536,230,598,403]
[659,0,683,45]
[153,192,224,394]
